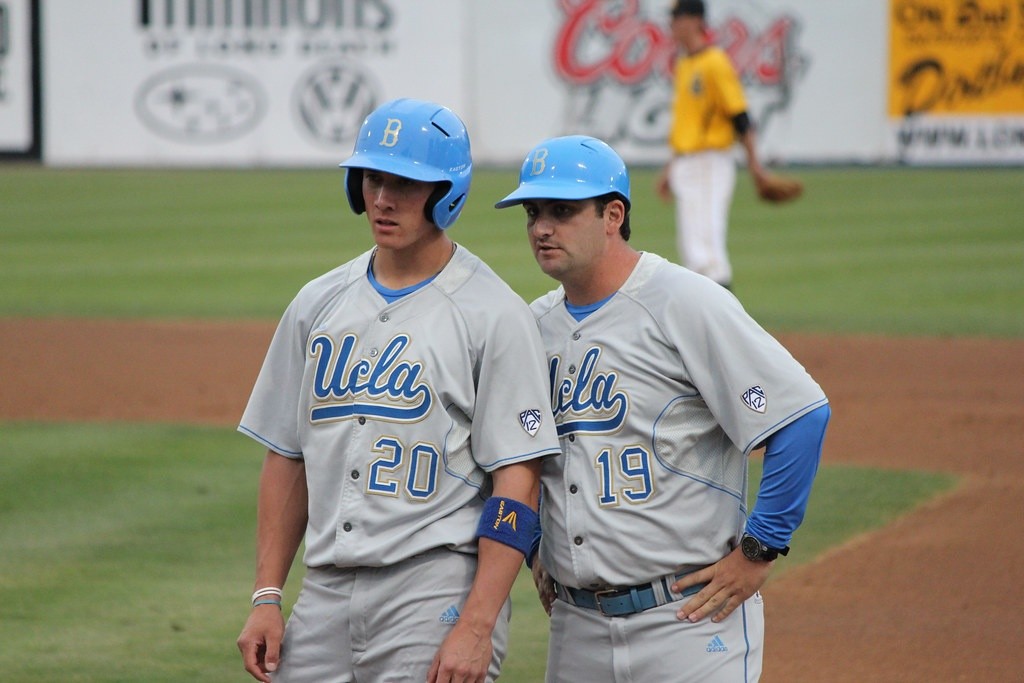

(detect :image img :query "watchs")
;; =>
[740,531,791,562]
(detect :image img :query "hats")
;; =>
[671,0,704,18]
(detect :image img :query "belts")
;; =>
[550,569,713,617]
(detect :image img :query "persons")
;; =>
[657,1,761,287]
[217,97,561,683]
[494,136,831,680]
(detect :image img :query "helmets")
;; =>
[495,136,631,210]
[339,98,472,229]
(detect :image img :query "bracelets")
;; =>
[254,600,280,607]
[252,587,282,605]
